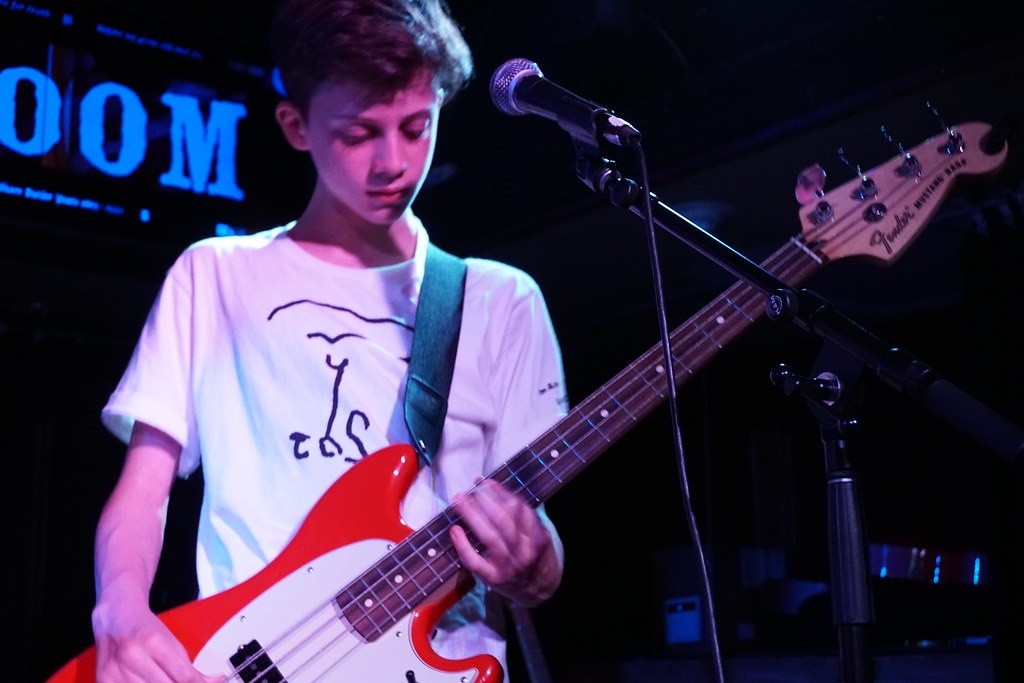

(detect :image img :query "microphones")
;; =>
[485,56,639,151]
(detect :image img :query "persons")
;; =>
[91,0,570,683]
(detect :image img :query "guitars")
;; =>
[42,89,1011,683]
